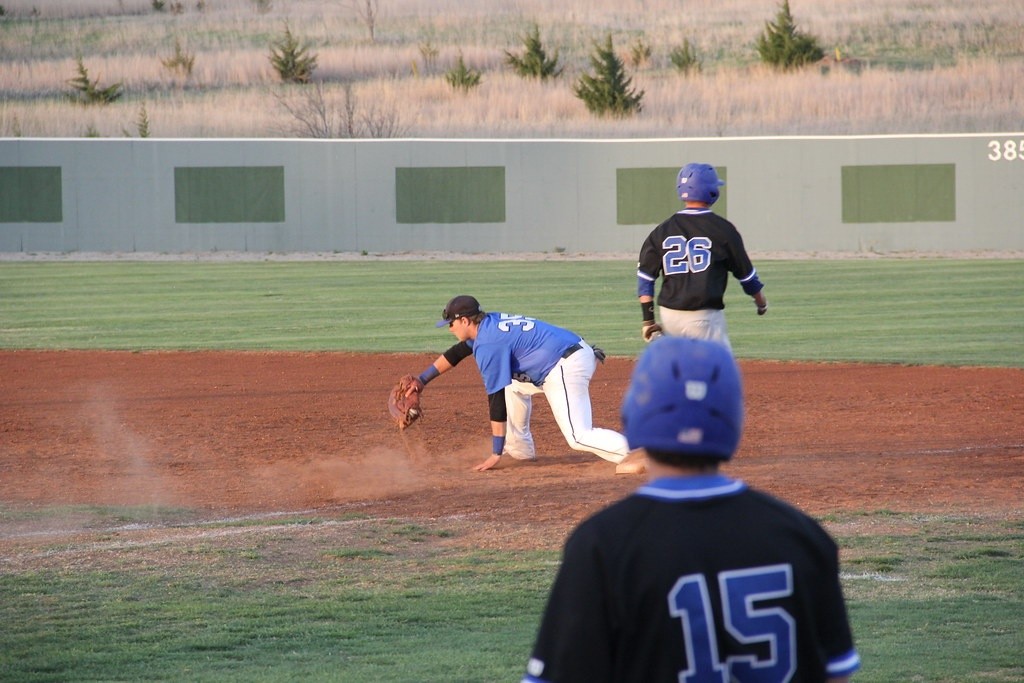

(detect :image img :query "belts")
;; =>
[562,344,583,358]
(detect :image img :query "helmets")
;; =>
[620,336,744,458]
[677,163,725,205]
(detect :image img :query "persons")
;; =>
[403,296,644,475]
[637,163,769,360]
[519,337,865,683]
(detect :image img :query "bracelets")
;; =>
[419,365,440,384]
[758,303,768,311]
[640,302,654,321]
[641,321,656,326]
[492,435,505,455]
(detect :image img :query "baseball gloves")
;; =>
[388,373,424,432]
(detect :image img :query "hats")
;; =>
[435,296,482,328]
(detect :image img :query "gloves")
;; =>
[642,320,666,343]
[758,301,768,317]
